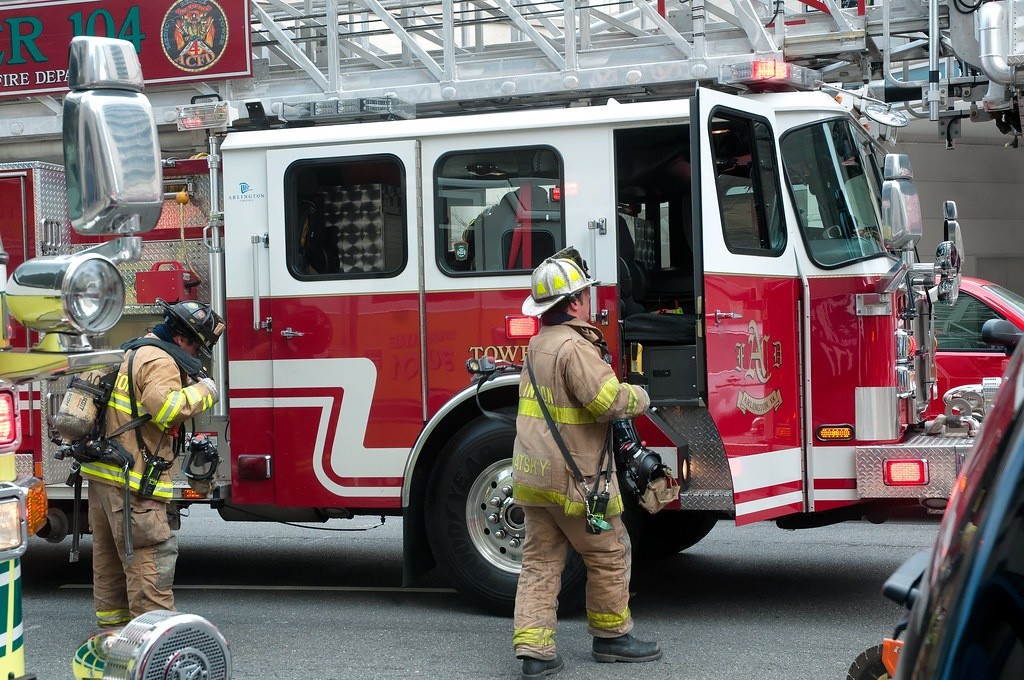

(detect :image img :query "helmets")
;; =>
[156,291,227,358]
[515,252,598,318]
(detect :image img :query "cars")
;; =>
[908,269,1024,418]
[827,326,1024,679]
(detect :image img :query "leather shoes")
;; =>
[591,629,664,665]
[517,655,564,680]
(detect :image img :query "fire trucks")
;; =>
[1,32,993,616]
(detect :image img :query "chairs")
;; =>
[617,213,698,343]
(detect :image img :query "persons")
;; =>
[510,252,670,678]
[83,299,227,629]
